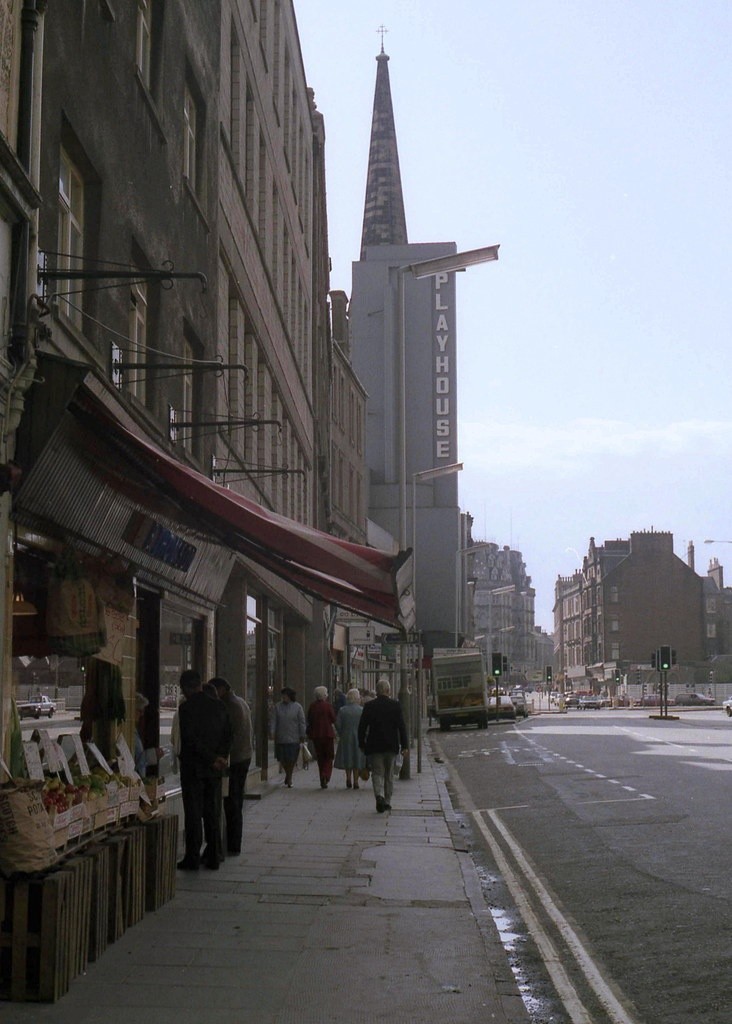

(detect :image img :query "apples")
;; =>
[45,768,128,814]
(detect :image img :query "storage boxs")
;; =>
[40,773,166,858]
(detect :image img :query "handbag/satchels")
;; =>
[359,767,369,781]
[302,743,312,764]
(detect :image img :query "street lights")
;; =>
[485,583,518,676]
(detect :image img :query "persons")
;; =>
[202,683,230,863]
[171,694,186,768]
[707,688,713,698]
[358,679,409,812]
[334,688,366,789]
[361,689,374,702]
[208,676,254,857]
[307,686,337,789]
[176,669,232,870]
[335,688,346,706]
[370,689,378,699]
[269,687,306,787]
[135,691,164,779]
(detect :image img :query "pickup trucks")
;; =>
[17,695,55,720]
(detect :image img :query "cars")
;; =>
[722,696,732,717]
[511,696,528,717]
[488,695,517,721]
[491,688,506,695]
[549,690,579,707]
[511,685,525,696]
[577,697,601,711]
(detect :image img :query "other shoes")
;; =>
[201,856,220,871]
[353,784,359,790]
[285,779,293,788]
[385,804,392,810]
[347,781,352,789]
[376,796,386,814]
[177,858,201,870]
[222,845,240,856]
[321,784,328,789]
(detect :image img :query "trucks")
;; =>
[431,647,490,731]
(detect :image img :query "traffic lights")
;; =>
[642,685,647,695]
[659,645,673,671]
[492,652,503,676]
[670,649,676,664]
[615,670,620,686]
[547,666,552,685]
[709,671,713,683]
[502,656,508,672]
[651,650,660,671]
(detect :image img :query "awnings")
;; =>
[88,398,417,632]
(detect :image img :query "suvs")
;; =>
[632,694,665,706]
[675,692,715,706]
